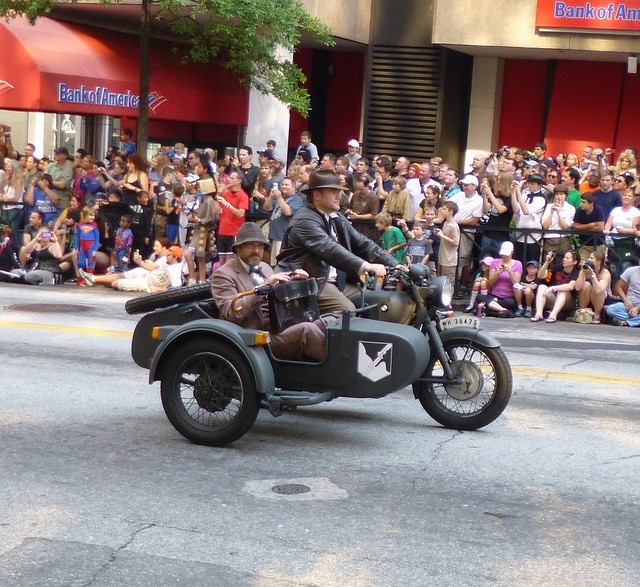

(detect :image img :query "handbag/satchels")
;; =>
[268,272,321,335]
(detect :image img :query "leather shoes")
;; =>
[463,305,474,313]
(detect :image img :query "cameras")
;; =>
[582,260,595,272]
[497,151,502,158]
[504,147,511,154]
[484,152,495,165]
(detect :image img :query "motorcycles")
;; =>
[124,263,513,444]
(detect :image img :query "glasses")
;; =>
[549,174,559,179]
[614,178,627,186]
[522,164,532,170]
[187,157,194,160]
[107,149,111,152]
[243,242,268,250]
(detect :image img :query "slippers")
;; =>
[592,317,601,324]
[546,315,557,323]
[530,314,544,322]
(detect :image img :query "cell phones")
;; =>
[596,154,604,158]
[607,149,615,153]
[549,253,556,258]
[97,168,102,174]
[483,176,490,189]
[556,197,561,206]
[273,183,278,193]
[37,178,41,187]
[501,265,504,272]
[435,229,440,235]
[40,231,52,238]
[179,164,185,175]
[513,176,521,187]
[233,147,238,160]
[135,249,139,260]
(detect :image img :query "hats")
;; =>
[231,221,272,253]
[554,183,568,192]
[300,169,350,194]
[67,210,81,222]
[544,158,554,167]
[523,160,540,171]
[480,256,494,266]
[257,150,273,157]
[625,172,634,180]
[348,138,360,147]
[409,163,420,177]
[95,191,107,201]
[526,259,540,269]
[162,246,183,259]
[54,146,69,155]
[527,173,548,186]
[499,241,514,257]
[459,174,479,187]
[299,147,311,163]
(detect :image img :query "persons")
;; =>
[44,146,74,209]
[74,149,106,206]
[541,184,576,266]
[211,222,338,361]
[252,161,279,223]
[131,191,153,259]
[561,145,640,213]
[441,175,483,297]
[174,140,232,183]
[383,175,412,221]
[345,176,380,243]
[604,188,640,278]
[510,174,547,265]
[414,185,445,224]
[513,260,540,318]
[463,256,494,315]
[476,241,523,318]
[14,143,49,174]
[573,191,607,264]
[165,183,184,245]
[480,172,514,260]
[149,143,175,215]
[257,140,285,183]
[607,266,640,327]
[396,206,440,279]
[100,153,148,233]
[106,214,134,273]
[430,155,461,198]
[185,177,220,287]
[530,250,581,323]
[405,221,434,268]
[78,245,183,293]
[231,146,258,196]
[0,132,26,283]
[74,205,100,287]
[466,143,561,184]
[19,208,45,267]
[105,146,118,163]
[145,238,170,262]
[54,194,82,245]
[433,201,460,314]
[215,171,249,252]
[25,226,71,281]
[287,131,320,201]
[26,174,59,230]
[375,211,408,291]
[56,210,81,283]
[274,169,409,316]
[394,156,420,178]
[575,251,612,324]
[320,139,393,200]
[99,163,128,195]
[120,129,136,153]
[403,161,444,221]
[262,177,304,267]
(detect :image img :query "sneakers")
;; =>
[437,307,454,315]
[498,309,515,317]
[524,310,533,318]
[514,309,525,317]
[612,317,626,326]
[79,268,95,287]
